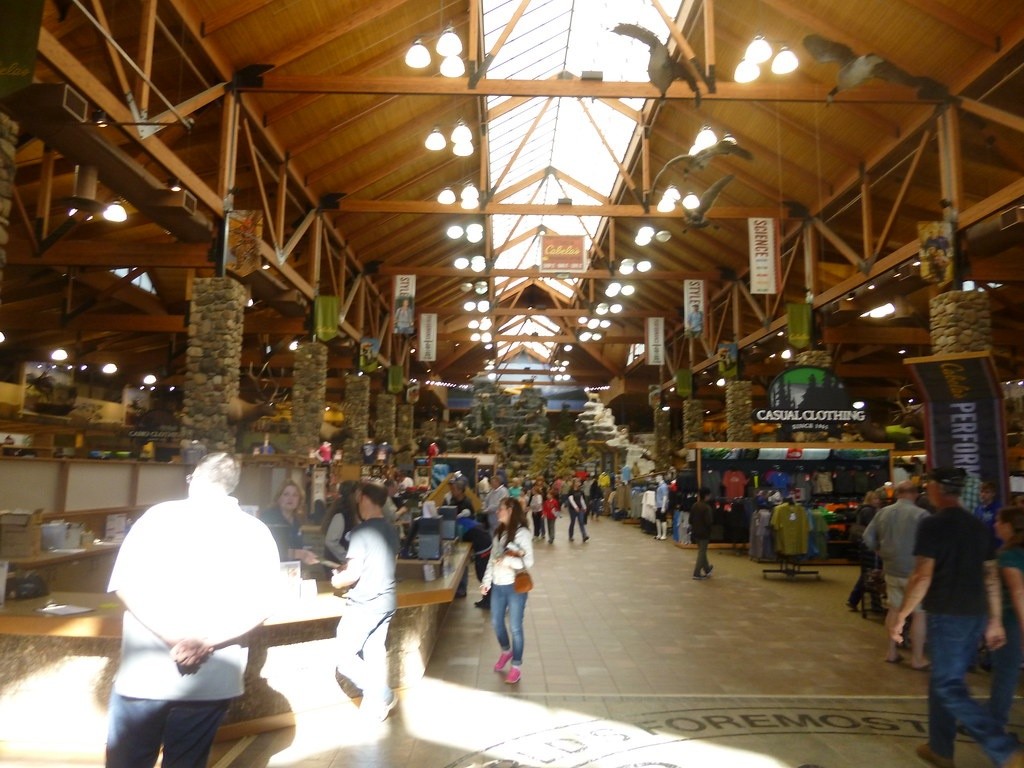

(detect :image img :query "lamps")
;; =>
[657,184,700,212]
[604,279,636,298]
[426,118,474,156]
[733,33,798,83]
[102,198,127,222]
[619,257,652,275]
[438,181,479,209]
[634,226,671,246]
[455,255,487,272]
[447,222,484,244]
[688,121,737,156]
[404,20,465,77]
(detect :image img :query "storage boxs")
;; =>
[0,508,44,557]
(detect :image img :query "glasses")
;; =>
[184,472,210,483]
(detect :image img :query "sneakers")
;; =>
[692,565,713,580]
[495,652,521,683]
[532,535,589,543]
[474,598,491,609]
[356,694,398,724]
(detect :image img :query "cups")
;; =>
[424,565,435,582]
[299,579,318,598]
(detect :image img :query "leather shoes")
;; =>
[917,744,955,768]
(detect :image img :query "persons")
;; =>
[651,475,669,541]
[257,465,1024,768]
[393,299,414,334]
[106,454,283,768]
[686,304,703,338]
[925,222,951,283]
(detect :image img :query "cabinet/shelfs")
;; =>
[0,456,188,593]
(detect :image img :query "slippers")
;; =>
[886,654,899,662]
[913,658,931,670]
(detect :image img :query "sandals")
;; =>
[845,599,859,612]
[872,607,888,616]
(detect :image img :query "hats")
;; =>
[448,470,468,483]
[929,464,965,487]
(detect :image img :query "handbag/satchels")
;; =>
[690,534,697,544]
[515,556,533,594]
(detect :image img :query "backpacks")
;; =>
[592,499,604,514]
